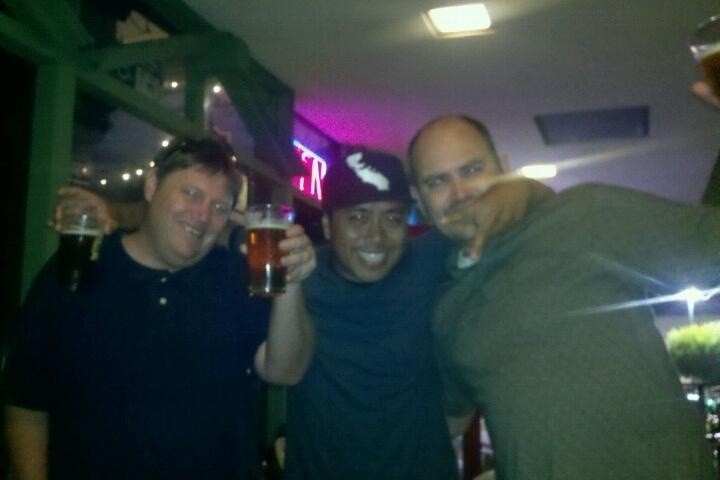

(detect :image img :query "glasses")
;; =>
[166,139,239,166]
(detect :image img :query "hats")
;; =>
[322,149,418,210]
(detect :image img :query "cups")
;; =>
[243,204,295,296]
[54,198,101,264]
[688,14,719,99]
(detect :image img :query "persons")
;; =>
[408,81,720,480]
[2,134,317,479]
[47,151,557,480]
